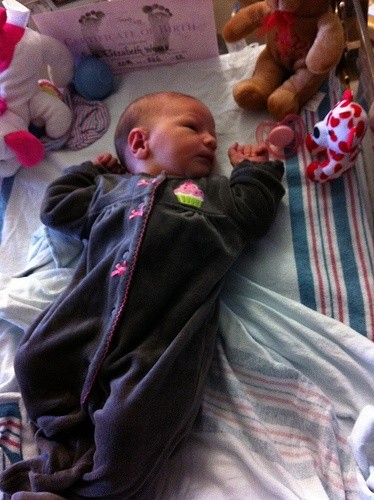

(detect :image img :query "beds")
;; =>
[0,0,374,500]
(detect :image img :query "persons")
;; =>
[0,91,285,500]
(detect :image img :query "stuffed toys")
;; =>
[223,0,345,121]
[304,89,368,183]
[1,6,75,178]
